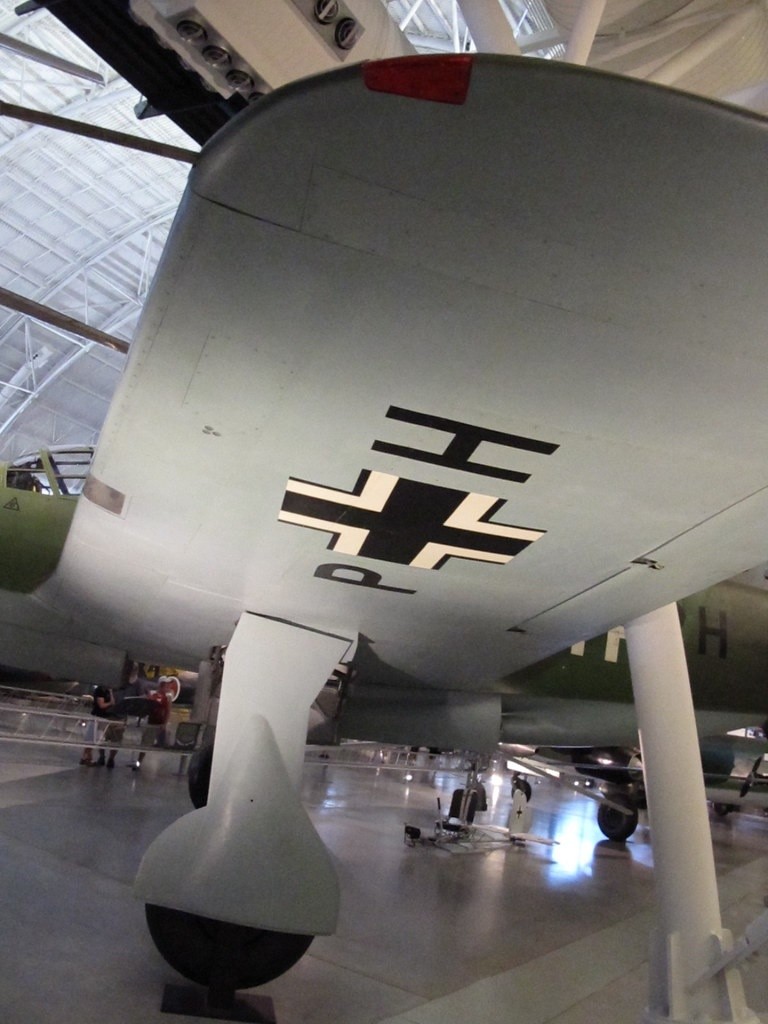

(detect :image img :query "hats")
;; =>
[157,675,174,686]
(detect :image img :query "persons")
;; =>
[94,661,145,769]
[127,676,171,771]
[79,685,114,766]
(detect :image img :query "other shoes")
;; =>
[86,759,95,765]
[95,756,106,766]
[127,760,136,767]
[106,758,114,768]
[80,759,90,765]
[132,761,142,771]
